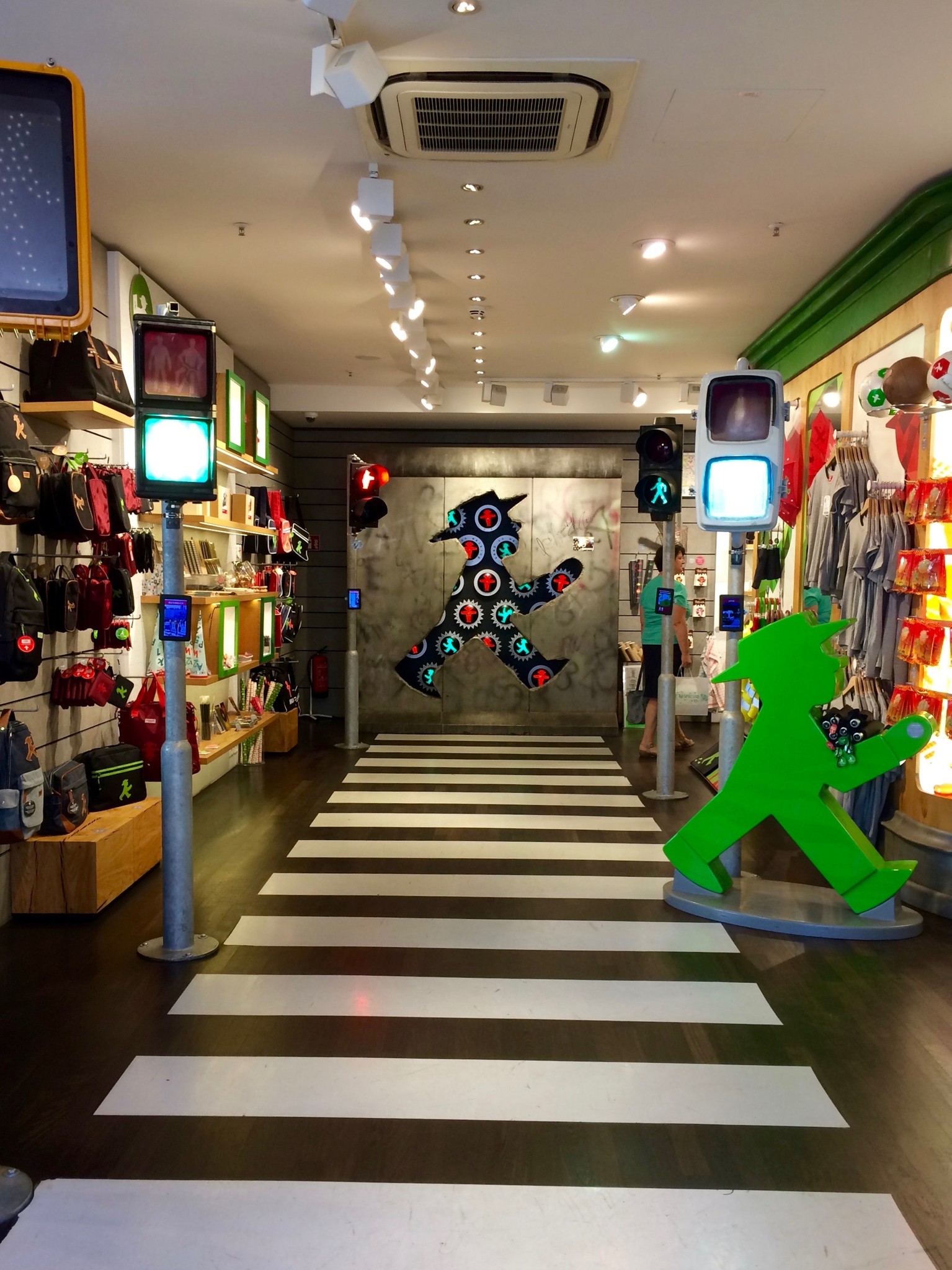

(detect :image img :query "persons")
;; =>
[640,543,695,757]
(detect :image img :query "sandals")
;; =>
[675,737,695,750]
[639,743,658,757]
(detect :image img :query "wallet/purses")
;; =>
[50,657,135,709]
[254,564,303,648]
[92,622,132,652]
[36,455,155,632]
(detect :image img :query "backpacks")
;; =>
[251,657,302,718]
[0,391,40,526]
[0,550,44,686]
[0,708,44,844]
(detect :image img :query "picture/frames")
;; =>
[260,596,274,662]
[254,390,269,466]
[226,369,245,454]
[218,599,238,678]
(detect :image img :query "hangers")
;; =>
[826,430,868,468]
[744,599,790,625]
[860,480,901,518]
[831,642,881,696]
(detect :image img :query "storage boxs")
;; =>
[263,708,298,752]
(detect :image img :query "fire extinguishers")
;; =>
[307,646,328,698]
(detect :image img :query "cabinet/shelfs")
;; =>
[20,401,279,764]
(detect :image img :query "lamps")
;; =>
[593,335,623,353]
[311,30,445,410]
[481,383,647,407]
[633,238,676,259]
[609,295,645,315]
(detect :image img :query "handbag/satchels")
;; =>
[675,665,709,716]
[29,326,135,417]
[37,759,90,835]
[242,486,311,562]
[626,690,649,724]
[113,670,201,781]
[73,745,147,812]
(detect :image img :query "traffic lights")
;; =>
[634,424,684,514]
[131,312,220,503]
[348,461,389,529]
[693,368,784,533]
